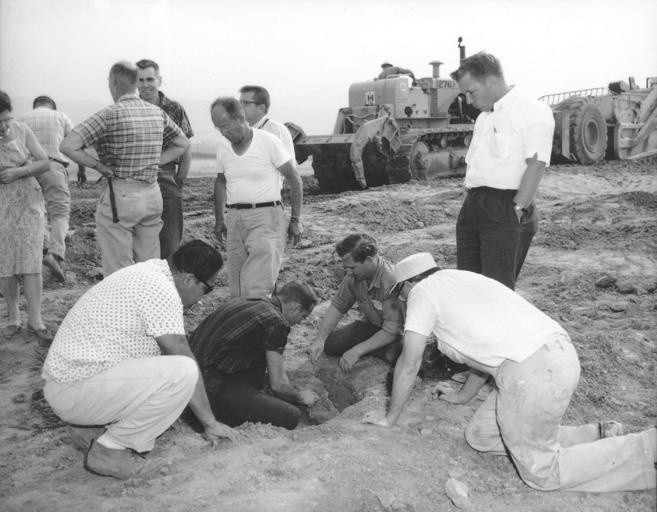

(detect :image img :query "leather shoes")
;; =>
[41,253,65,283]
[3,324,20,338]
[26,319,53,341]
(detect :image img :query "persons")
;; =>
[58,60,190,280]
[450,51,556,292]
[133,60,195,261]
[40,240,239,480]
[187,281,320,430]
[361,251,657,493]
[209,95,304,298]
[302,233,411,372]
[15,95,86,284]
[238,84,296,193]
[1,91,55,343]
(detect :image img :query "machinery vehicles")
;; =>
[536,76,656,167]
[280,36,480,190]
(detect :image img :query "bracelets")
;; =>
[289,216,300,224]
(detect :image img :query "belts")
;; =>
[226,201,281,209]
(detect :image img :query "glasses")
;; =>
[394,279,412,302]
[240,99,255,105]
[187,270,213,295]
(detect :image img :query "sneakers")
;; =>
[599,419,624,439]
[86,438,166,481]
[64,424,98,455]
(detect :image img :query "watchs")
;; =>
[509,199,531,215]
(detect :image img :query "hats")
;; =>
[388,252,438,296]
